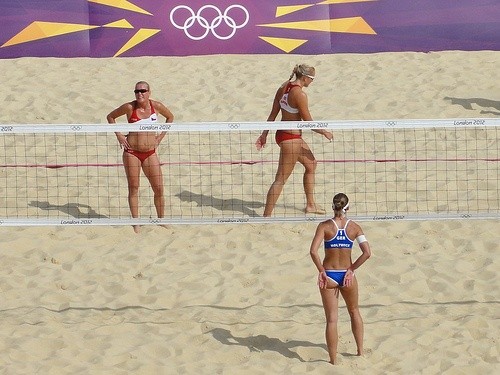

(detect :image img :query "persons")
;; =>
[310,194,371,365]
[107,81,174,233]
[255,64,334,217]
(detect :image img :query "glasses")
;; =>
[134,89,148,94]
[302,73,315,80]
